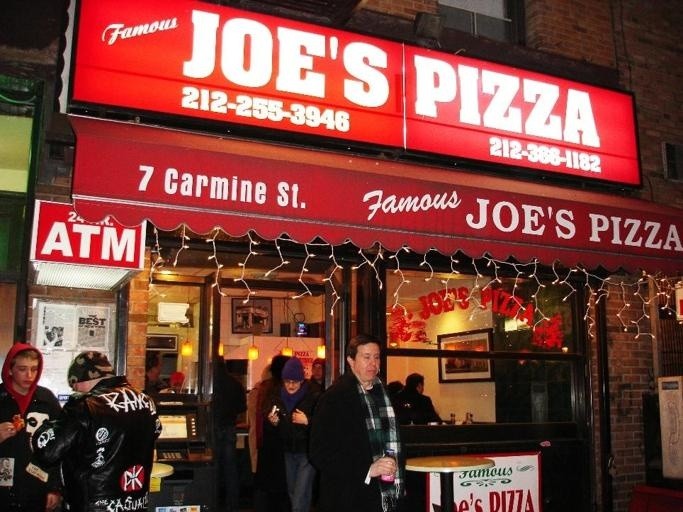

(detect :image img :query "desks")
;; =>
[403,455,495,511]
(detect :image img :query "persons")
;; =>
[145,352,175,393]
[0,342,65,512]
[44,351,161,512]
[216,353,443,511]
[307,334,410,511]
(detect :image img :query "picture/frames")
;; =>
[437,327,495,384]
[231,298,273,334]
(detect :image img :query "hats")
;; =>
[281,356,305,381]
[67,351,116,388]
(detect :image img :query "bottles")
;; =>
[379,449,397,489]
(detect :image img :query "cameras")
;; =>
[271,404,289,418]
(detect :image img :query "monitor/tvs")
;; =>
[157,414,188,439]
